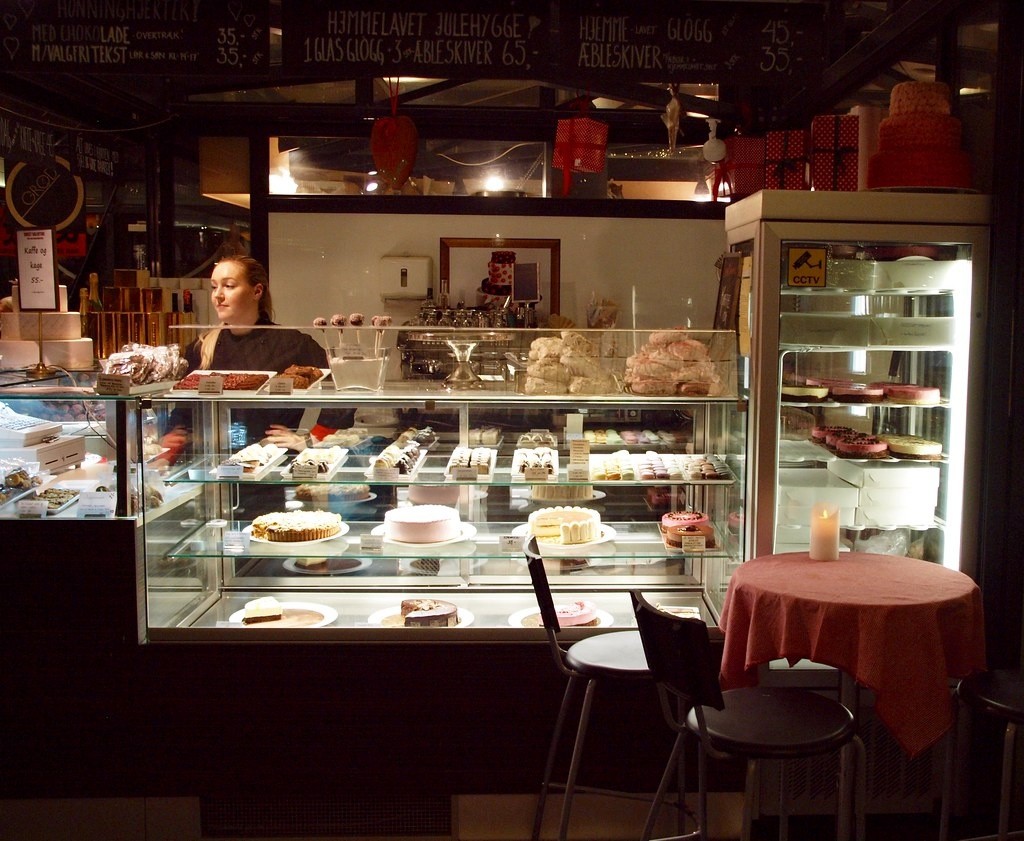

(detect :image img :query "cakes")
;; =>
[294,557,587,571]
[726,512,740,535]
[782,376,943,460]
[662,511,715,549]
[251,510,342,542]
[476,250,516,309]
[242,596,597,627]
[527,506,602,544]
[385,505,461,543]
[294,483,593,505]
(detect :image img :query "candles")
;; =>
[810,501,840,561]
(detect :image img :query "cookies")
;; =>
[0,467,164,513]
[593,449,732,480]
[584,429,688,446]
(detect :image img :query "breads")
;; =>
[108,353,324,390]
[525,332,721,397]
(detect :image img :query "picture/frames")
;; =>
[440,237,559,317]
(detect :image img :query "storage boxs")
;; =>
[733,134,765,195]
[87,268,195,360]
[765,130,806,189]
[552,118,608,175]
[811,112,860,191]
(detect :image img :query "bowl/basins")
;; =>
[830,245,874,290]
[324,344,391,392]
[874,245,958,287]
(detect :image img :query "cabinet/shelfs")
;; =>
[0,370,746,799]
[726,220,991,613]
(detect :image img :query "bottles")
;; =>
[88,273,103,340]
[474,304,533,328]
[438,279,450,308]
[408,307,476,328]
[166,292,197,358]
[421,287,435,312]
[80,288,89,338]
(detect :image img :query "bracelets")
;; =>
[305,434,313,449]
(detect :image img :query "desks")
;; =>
[720,550,986,841]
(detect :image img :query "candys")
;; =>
[313,313,392,326]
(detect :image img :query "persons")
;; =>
[148,257,357,574]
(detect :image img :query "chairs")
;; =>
[628,587,869,841]
[521,533,688,841]
[951,650,1024,841]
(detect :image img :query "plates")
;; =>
[243,521,350,546]
[282,558,372,574]
[516,432,692,450]
[589,454,736,485]
[371,522,477,547]
[399,558,487,575]
[229,603,338,627]
[396,489,489,501]
[364,449,560,479]
[313,434,504,448]
[286,492,377,504]
[280,448,349,478]
[512,523,617,548]
[508,607,614,627]
[368,607,476,628]
[209,444,288,476]
[520,490,606,505]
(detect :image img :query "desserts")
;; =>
[468,426,554,448]
[448,448,554,473]
[38,402,108,420]
[398,427,435,448]
[223,443,421,474]
